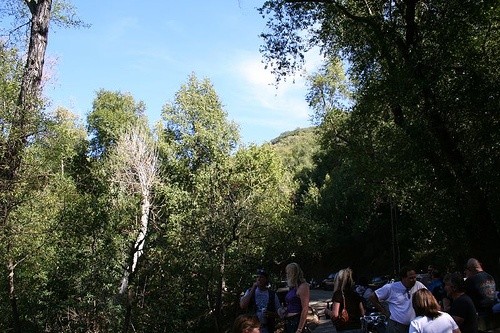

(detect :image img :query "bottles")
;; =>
[326,302,331,319]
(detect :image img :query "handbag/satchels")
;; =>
[333,289,349,329]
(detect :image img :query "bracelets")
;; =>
[297,328,302,332]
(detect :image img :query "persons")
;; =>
[283,262,310,333]
[443,272,479,333]
[240,269,283,333]
[324,269,365,333]
[420,269,500,333]
[408,288,462,333]
[369,267,428,333]
[464,258,498,333]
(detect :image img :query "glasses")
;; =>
[257,268,266,273]
